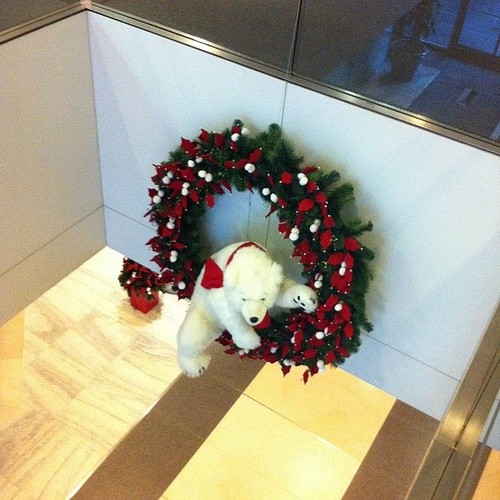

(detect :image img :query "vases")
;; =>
[130,282,158,314]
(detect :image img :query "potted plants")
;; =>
[389,0,441,82]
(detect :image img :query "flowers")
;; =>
[118,256,157,301]
[145,110,377,384]
